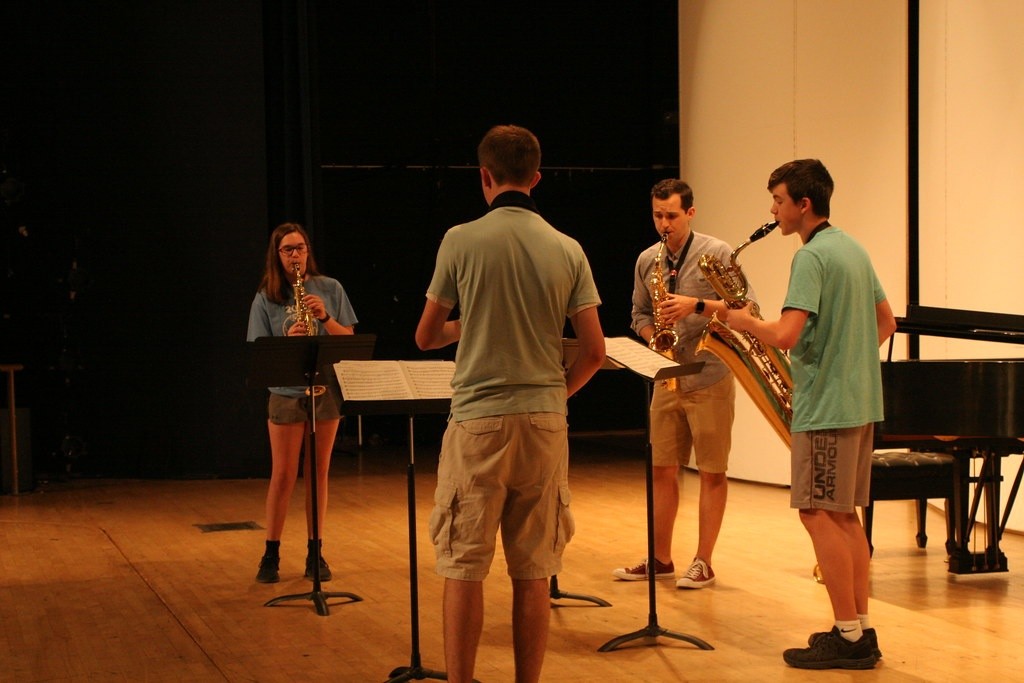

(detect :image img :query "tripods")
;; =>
[255,335,376,617]
[326,363,482,683]
[548,338,612,607]
[597,337,717,653]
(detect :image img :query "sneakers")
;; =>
[676,557,715,589]
[808,628,882,660]
[782,624,877,670]
[612,555,675,581]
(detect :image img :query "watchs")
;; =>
[694,296,707,317]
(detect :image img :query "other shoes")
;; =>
[255,555,280,583]
[305,551,332,581]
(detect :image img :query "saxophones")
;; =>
[647,232,679,353]
[695,221,795,453]
[291,261,326,396]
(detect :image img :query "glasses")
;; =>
[278,244,309,256]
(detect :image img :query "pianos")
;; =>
[870,298,1024,449]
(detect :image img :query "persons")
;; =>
[414,126,605,683]
[613,180,753,589]
[247,223,358,584]
[726,159,897,670]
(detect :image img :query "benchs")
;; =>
[859,452,958,562]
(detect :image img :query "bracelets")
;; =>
[318,311,332,324]
